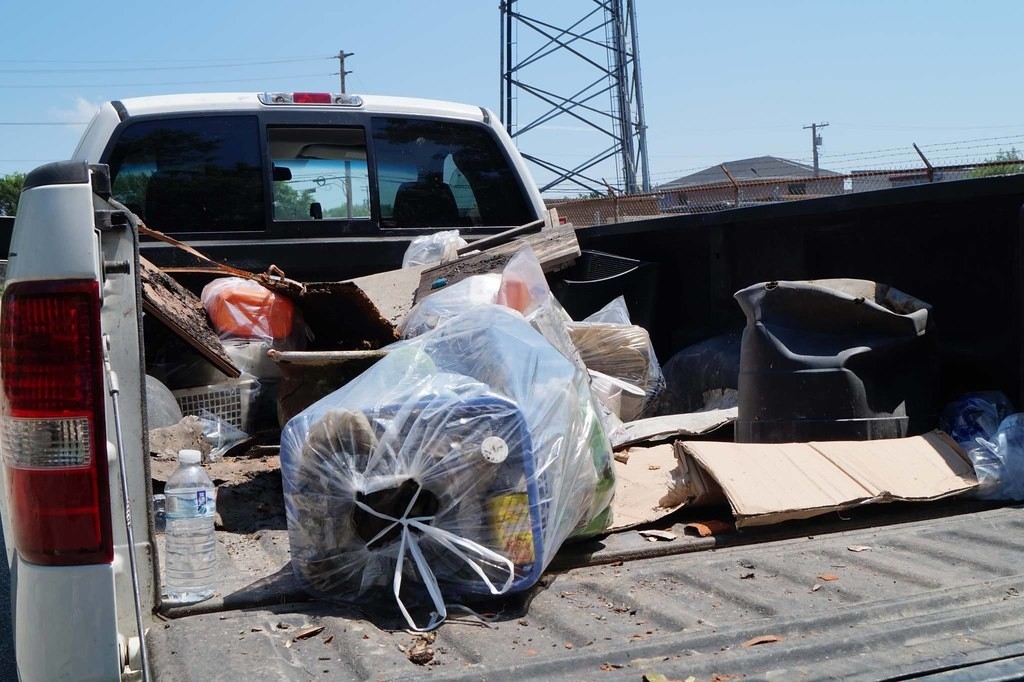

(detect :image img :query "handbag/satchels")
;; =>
[201,275,315,380]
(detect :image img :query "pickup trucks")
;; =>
[0,90,1024,681]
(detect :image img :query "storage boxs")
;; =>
[168,376,260,435]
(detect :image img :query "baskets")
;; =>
[555,245,643,286]
[281,389,553,599]
[171,376,255,445]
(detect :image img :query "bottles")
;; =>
[162,448,218,607]
[485,493,536,569]
[463,437,510,494]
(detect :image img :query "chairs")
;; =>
[391,183,461,227]
[146,163,275,239]
